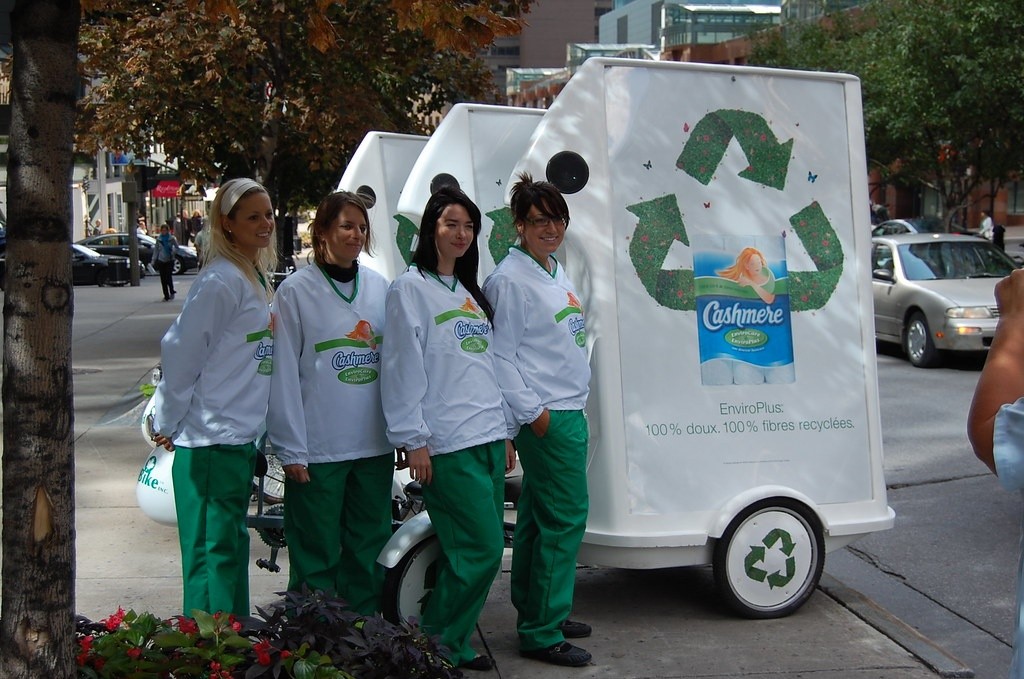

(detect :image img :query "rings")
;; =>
[304,467,306,470]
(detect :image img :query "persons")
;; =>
[482,171,591,668]
[136,209,147,235]
[103,229,118,245]
[266,191,409,617]
[380,188,520,679]
[151,224,179,302]
[93,219,100,235]
[967,264,1024,679]
[979,210,994,241]
[153,177,275,627]
[189,211,210,271]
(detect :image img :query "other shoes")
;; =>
[447,668,463,679]
[459,655,496,671]
[557,619,591,638]
[170,293,175,299]
[162,298,169,302]
[518,642,592,667]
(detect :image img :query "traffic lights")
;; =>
[136,163,161,193]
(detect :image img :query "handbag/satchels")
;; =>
[153,261,161,271]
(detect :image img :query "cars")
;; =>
[870,216,979,236]
[870,231,1022,368]
[0,243,146,291]
[74,232,201,275]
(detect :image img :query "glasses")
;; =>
[523,210,568,227]
